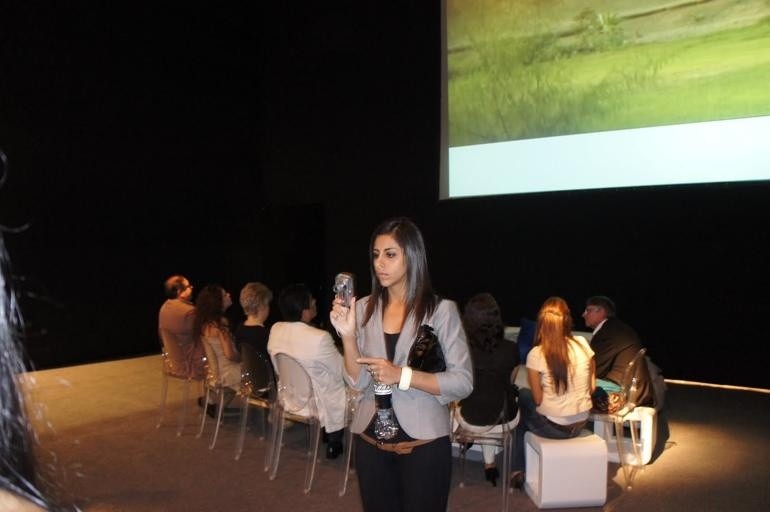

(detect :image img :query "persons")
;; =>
[510,296,596,490]
[158,274,236,422]
[582,296,657,414]
[194,284,242,391]
[454,294,522,486]
[266,284,349,459]
[0,237,65,512]
[234,280,278,402]
[330,214,474,511]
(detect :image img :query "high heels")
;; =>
[510,470,527,491]
[485,466,499,487]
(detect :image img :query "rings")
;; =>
[371,372,375,377]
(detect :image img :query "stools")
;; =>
[590,401,660,466]
[518,424,609,507]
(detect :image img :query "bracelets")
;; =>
[397,366,412,391]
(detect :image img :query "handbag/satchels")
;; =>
[407,325,446,372]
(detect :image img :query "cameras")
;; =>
[332,272,354,308]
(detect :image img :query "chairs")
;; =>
[152,325,204,436]
[335,379,364,494]
[195,330,268,451]
[446,367,513,510]
[582,343,649,491]
[261,349,340,493]
[231,340,313,470]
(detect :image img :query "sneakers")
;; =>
[325,442,343,459]
[198,396,218,419]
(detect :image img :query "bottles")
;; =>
[373,378,397,441]
[628,377,638,406]
